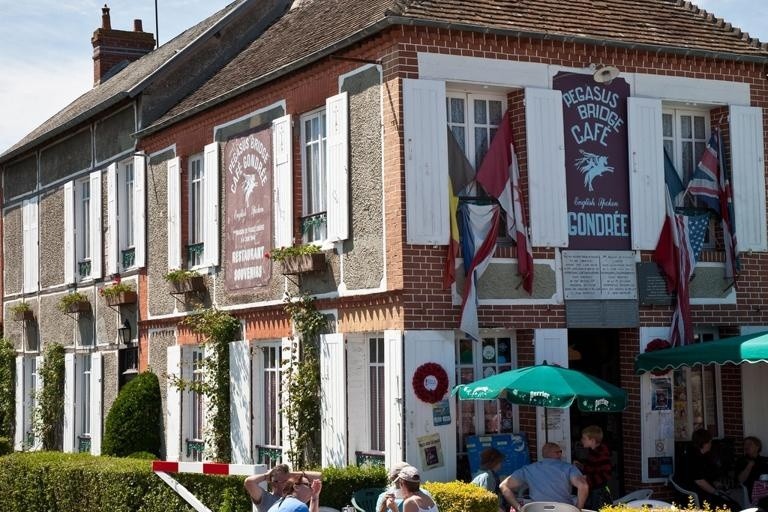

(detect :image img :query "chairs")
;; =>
[519,473,754,511]
[349,487,383,512]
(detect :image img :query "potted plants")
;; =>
[158,265,204,294]
[7,291,92,321]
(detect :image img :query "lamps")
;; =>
[553,63,620,84]
[117,317,133,349]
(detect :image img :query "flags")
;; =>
[651,126,741,349]
[446,110,535,341]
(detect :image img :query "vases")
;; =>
[278,253,325,275]
[105,292,136,307]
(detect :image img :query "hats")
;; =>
[394,466,420,483]
[481,449,504,469]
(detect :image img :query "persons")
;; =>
[267,473,322,512]
[466,447,506,512]
[499,441,589,512]
[739,436,767,510]
[375,461,432,512]
[245,463,322,512]
[572,425,613,509]
[672,429,741,511]
[394,466,440,512]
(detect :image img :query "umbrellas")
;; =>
[633,330,768,376]
[449,360,629,443]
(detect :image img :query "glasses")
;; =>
[300,482,311,488]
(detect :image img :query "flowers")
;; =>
[264,244,321,264]
[97,275,136,300]
